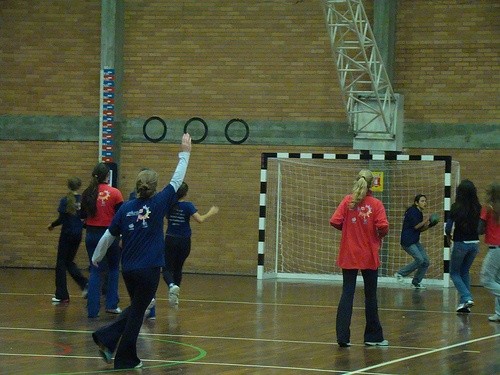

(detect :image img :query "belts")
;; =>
[489,246,500,249]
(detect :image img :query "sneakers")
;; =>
[364,339,388,345]
[410,282,425,288]
[133,362,143,368]
[168,284,180,305]
[337,340,350,346]
[92,331,113,363]
[488,312,500,321]
[394,272,405,285]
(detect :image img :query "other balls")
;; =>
[428,214,440,224]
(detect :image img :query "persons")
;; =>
[477,184,500,321]
[393,194,438,289]
[330,169,390,348]
[445,180,481,314]
[47,177,89,304]
[79,132,191,370]
[162,182,219,305]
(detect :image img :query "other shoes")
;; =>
[88,315,99,322]
[456,304,470,312]
[106,307,122,313]
[82,283,89,297]
[52,297,70,301]
[463,301,474,308]
[145,298,156,316]
[149,317,155,320]
